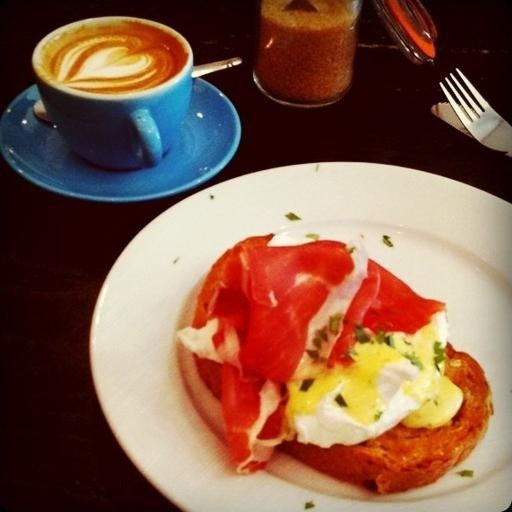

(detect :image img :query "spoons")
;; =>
[35,51,242,125]
[438,62,512,155]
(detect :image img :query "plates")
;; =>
[2,77,240,200]
[90,158,512,512]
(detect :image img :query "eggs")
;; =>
[178,299,464,449]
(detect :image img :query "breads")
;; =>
[276,341,494,496]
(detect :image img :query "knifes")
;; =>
[430,100,481,137]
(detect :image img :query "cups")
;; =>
[247,0,358,108]
[33,14,199,170]
